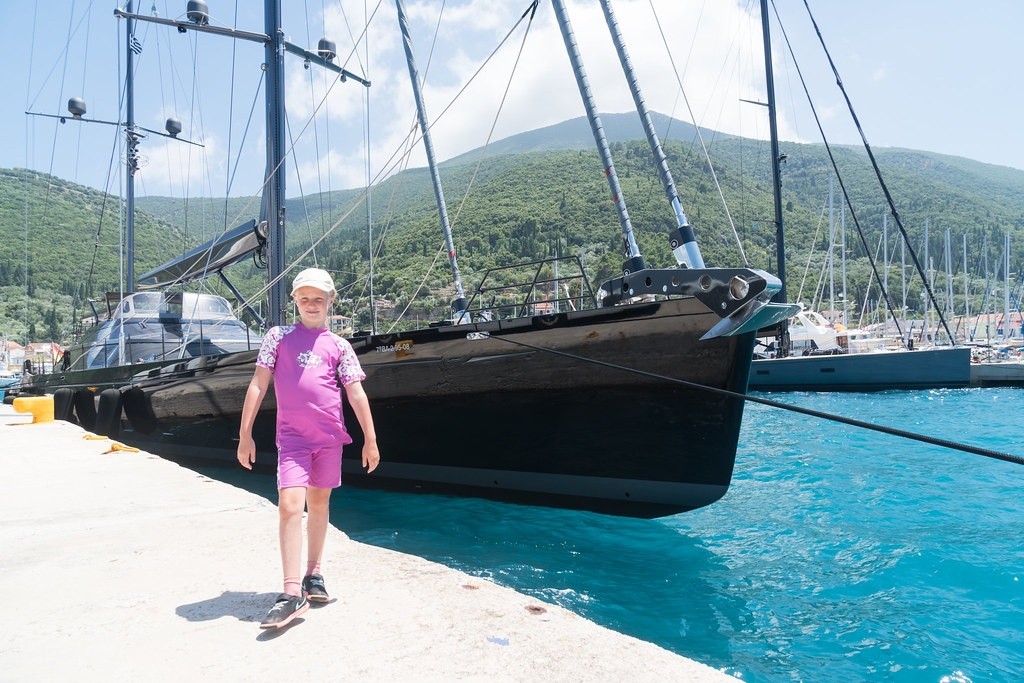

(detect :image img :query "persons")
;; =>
[62,348,72,372]
[237,268,381,632]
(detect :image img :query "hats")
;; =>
[290,268,334,296]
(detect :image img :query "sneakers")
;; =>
[302,573,329,603]
[260,593,310,629]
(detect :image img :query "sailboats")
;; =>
[410,0,1022,389]
[0,2,806,518]
